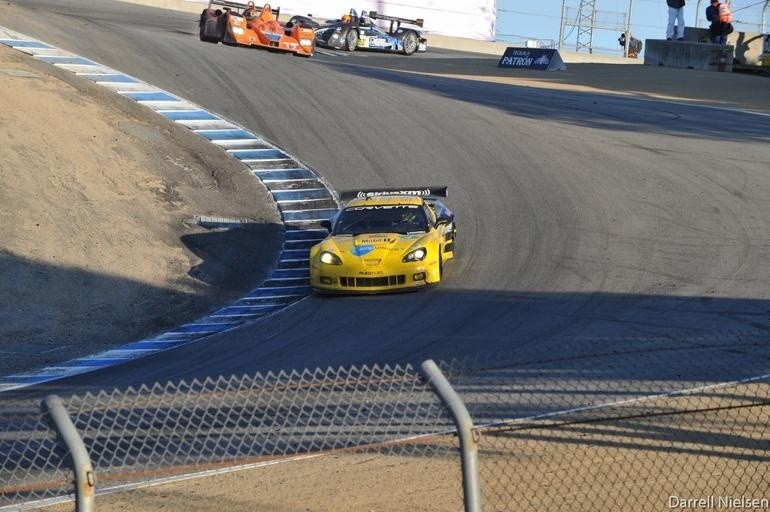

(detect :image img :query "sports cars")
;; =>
[307,185,458,295]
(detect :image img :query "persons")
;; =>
[665,0,686,41]
[706,0,734,44]
[620,32,643,58]
[389,208,419,231]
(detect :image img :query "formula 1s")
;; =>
[199,0,316,60]
[289,7,427,56]
